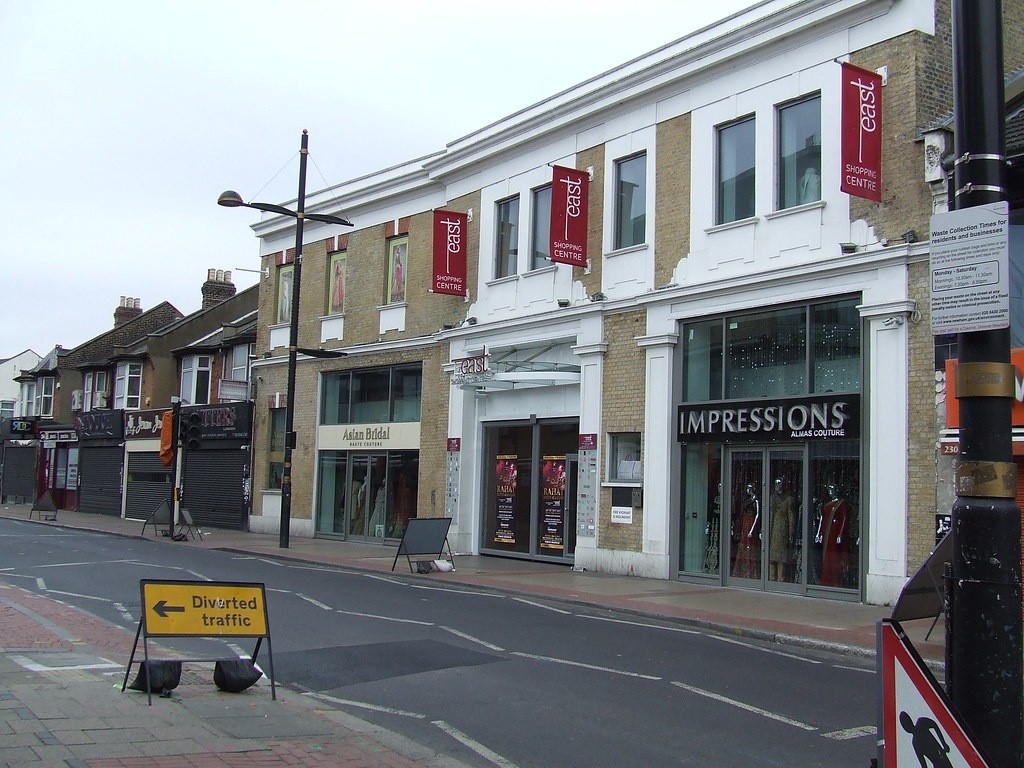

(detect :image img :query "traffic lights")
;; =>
[178,418,189,447]
[187,407,202,450]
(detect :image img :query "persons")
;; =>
[393,245,404,300]
[332,261,343,312]
[703,478,848,587]
[281,273,291,324]
[341,474,413,538]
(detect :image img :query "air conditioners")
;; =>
[96,391,107,407]
[72,390,83,410]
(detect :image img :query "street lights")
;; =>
[216,130,356,548]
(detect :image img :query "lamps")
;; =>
[443,323,452,329]
[591,292,603,300]
[839,243,857,254]
[557,299,569,307]
[263,352,272,357]
[901,230,918,243]
[249,355,258,360]
[465,317,477,325]
